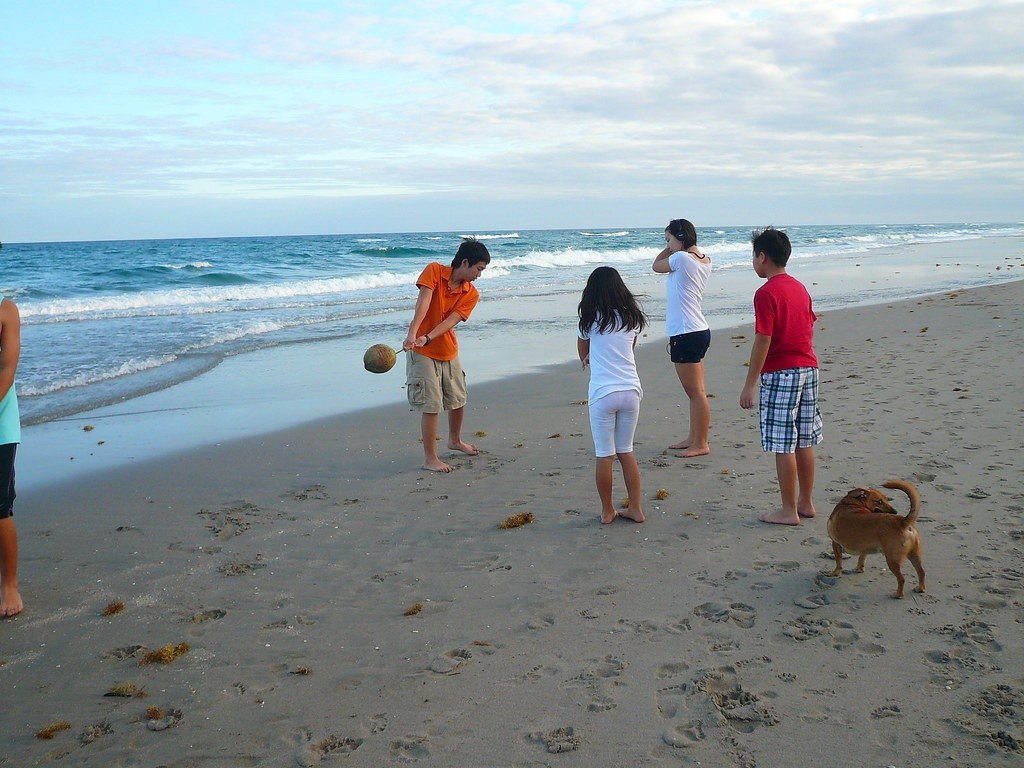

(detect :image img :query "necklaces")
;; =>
[688,250,706,259]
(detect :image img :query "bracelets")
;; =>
[423,335,430,347]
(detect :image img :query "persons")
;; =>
[652,219,711,459]
[402,236,491,474]
[739,224,824,525]
[0,241,24,619]
[578,266,646,523]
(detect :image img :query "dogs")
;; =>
[826,479,928,600]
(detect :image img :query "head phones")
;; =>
[676,219,685,241]
